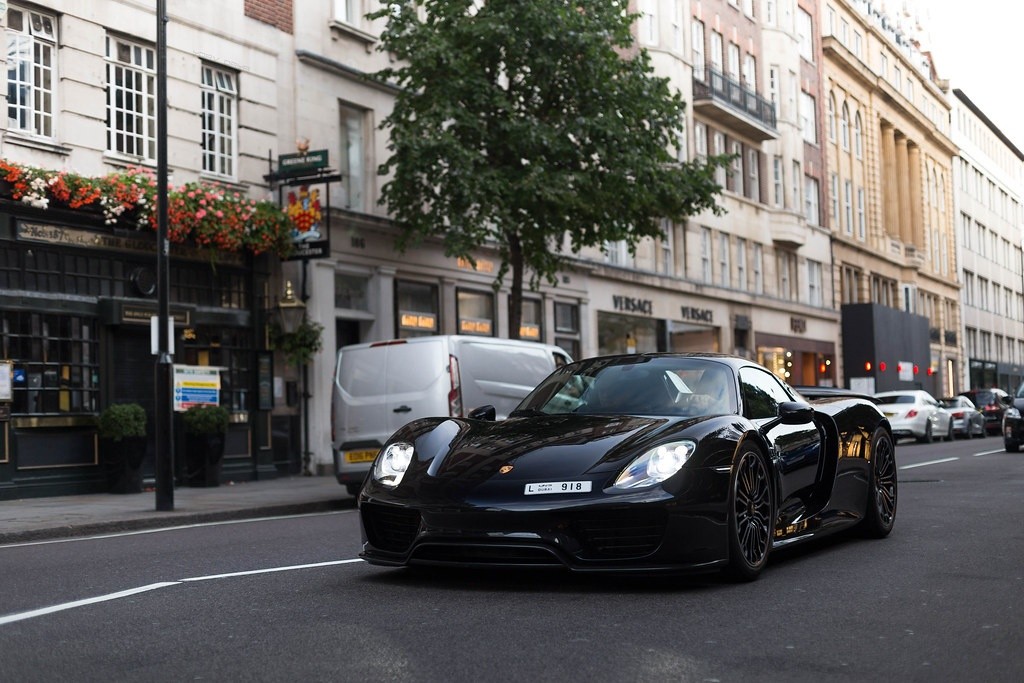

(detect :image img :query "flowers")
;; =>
[0,157,297,256]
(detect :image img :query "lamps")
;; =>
[256,279,304,337]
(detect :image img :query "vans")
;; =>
[332,334,591,497]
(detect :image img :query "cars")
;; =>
[872,390,954,444]
[957,388,1008,435]
[935,395,987,442]
[1001,381,1024,453]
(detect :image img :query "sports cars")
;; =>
[356,353,898,580]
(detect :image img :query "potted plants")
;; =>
[182,404,229,487]
[94,402,147,495]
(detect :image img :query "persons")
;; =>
[220,372,232,410]
